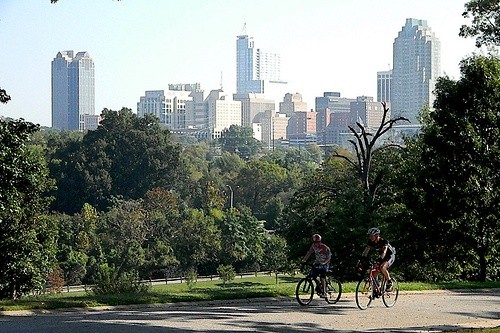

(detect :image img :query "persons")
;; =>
[359,228,395,299]
[302,234,331,297]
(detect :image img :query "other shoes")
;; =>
[385,282,393,292]
[321,293,328,297]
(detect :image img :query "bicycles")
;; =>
[295,262,342,306]
[354,264,400,311]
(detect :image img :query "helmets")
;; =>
[366,228,380,235]
[311,234,322,242]
